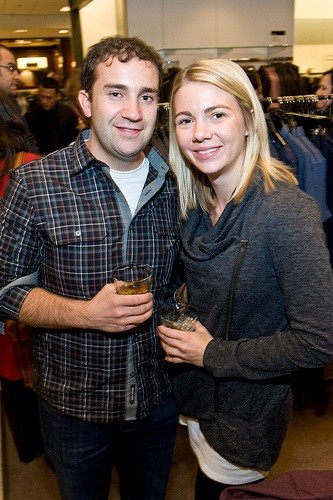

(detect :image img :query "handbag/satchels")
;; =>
[177,364,220,422]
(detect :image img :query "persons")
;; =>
[157,61,333,500]
[0,36,184,500]
[0,47,333,467]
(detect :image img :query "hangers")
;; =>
[160,59,179,76]
[233,56,293,74]
[264,93,333,164]
[149,101,170,164]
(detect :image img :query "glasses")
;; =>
[0,65,22,74]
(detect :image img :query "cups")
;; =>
[159,303,198,358]
[112,263,152,296]
